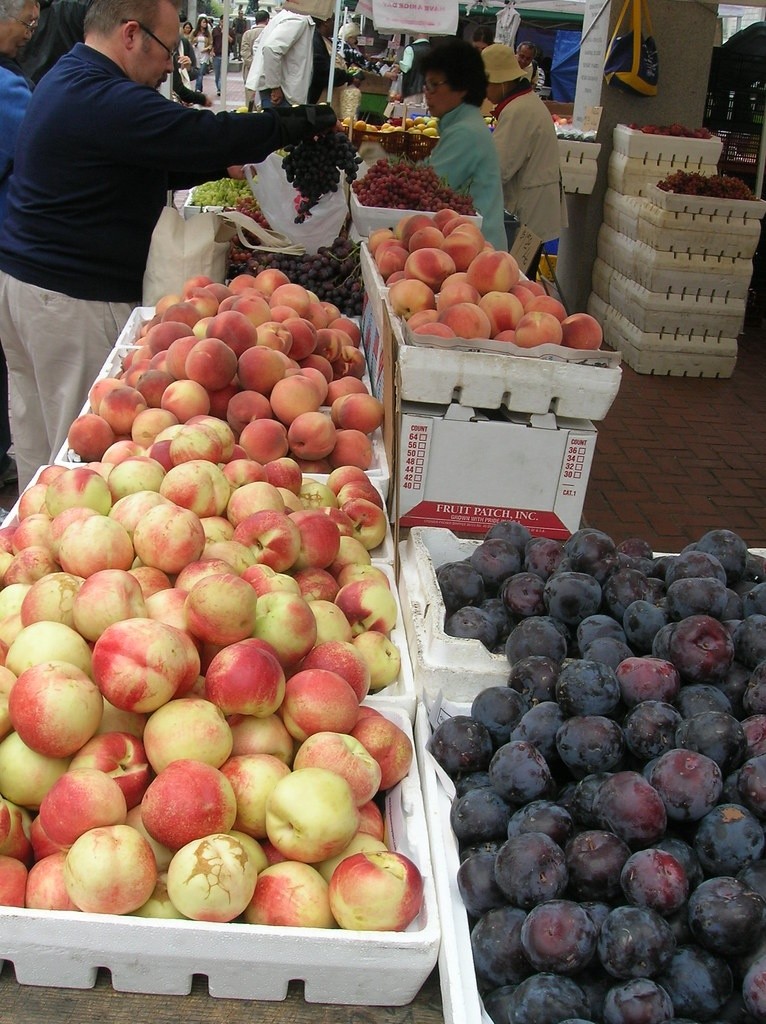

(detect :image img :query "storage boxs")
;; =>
[0,242,765,1024]
[349,182,483,245]
[343,69,601,194]
[588,122,766,379]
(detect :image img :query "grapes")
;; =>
[350,158,478,217]
[192,131,366,319]
[657,168,760,201]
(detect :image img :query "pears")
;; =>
[342,115,496,157]
[236,107,249,113]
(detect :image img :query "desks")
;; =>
[358,93,388,123]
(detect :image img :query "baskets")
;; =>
[341,125,440,163]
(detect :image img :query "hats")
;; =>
[481,44,529,83]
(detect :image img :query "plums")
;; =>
[628,122,710,138]
[435,520,766,1024]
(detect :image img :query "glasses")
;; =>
[422,80,448,93]
[120,18,177,59]
[8,15,38,30]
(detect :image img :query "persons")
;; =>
[172,11,248,96]
[244,9,316,110]
[335,21,398,81]
[306,13,360,107]
[390,33,430,102]
[417,36,508,255]
[1,0,348,497]
[482,43,569,282]
[514,41,545,98]
[0,0,40,513]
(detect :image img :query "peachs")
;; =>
[551,113,573,125]
[369,209,602,351]
[69,270,384,470]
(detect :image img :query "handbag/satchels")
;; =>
[388,73,404,103]
[603,31,660,97]
[142,203,307,308]
[244,152,348,255]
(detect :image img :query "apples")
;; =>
[0,417,421,933]
